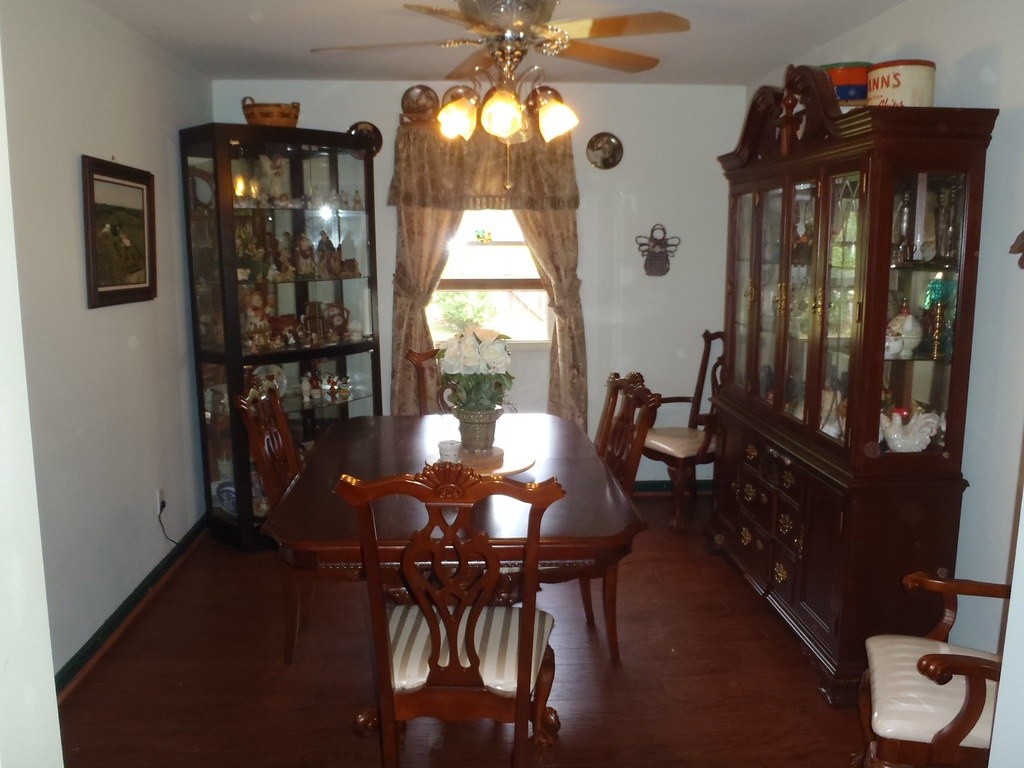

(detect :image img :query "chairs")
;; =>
[337,461,566,767]
[856,570,1012,767]
[405,346,456,415]
[508,367,665,659]
[239,371,369,662]
[631,328,726,533]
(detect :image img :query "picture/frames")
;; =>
[81,154,158,309]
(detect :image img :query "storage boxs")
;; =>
[866,59,936,107]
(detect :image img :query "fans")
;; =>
[309,1,691,82]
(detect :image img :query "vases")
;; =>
[449,403,504,453]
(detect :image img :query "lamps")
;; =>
[436,34,582,143]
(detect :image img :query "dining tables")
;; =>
[260,413,649,734]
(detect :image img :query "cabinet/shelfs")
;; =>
[179,119,382,553]
[703,63,1004,711]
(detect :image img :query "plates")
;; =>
[216,482,237,516]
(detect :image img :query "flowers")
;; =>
[433,324,513,412]
